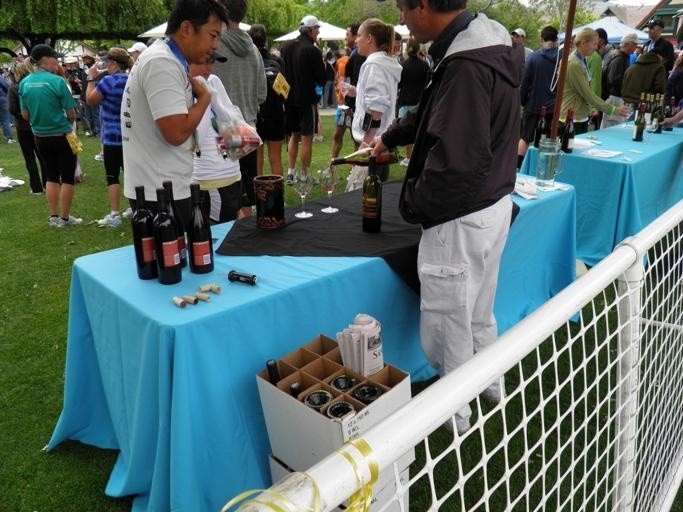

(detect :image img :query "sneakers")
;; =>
[399,156,411,167]
[121,208,134,218]
[93,153,105,161]
[48,214,84,227]
[28,187,48,197]
[285,171,321,187]
[96,213,120,227]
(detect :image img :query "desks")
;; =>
[518,115,682,269]
[39,169,578,489]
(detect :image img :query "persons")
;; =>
[1,42,150,228]
[554,21,682,137]
[120,3,336,232]
[370,2,525,439]
[325,3,559,196]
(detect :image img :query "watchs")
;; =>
[87,75,96,81]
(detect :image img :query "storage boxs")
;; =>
[252,332,418,507]
[267,456,409,512]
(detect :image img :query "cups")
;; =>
[537,138,564,187]
[253,174,285,229]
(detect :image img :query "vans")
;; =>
[642,6,682,36]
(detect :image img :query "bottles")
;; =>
[534,104,550,149]
[561,109,576,154]
[330,149,398,167]
[632,102,646,142]
[639,92,675,134]
[361,156,383,232]
[132,180,214,284]
[264,357,382,423]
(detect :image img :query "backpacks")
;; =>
[260,56,289,124]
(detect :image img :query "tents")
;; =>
[557,16,653,48]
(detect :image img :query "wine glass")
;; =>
[293,168,312,219]
[317,167,340,214]
[339,76,350,110]
[622,103,633,128]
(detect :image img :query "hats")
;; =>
[511,26,528,40]
[101,53,131,64]
[298,13,324,33]
[619,31,647,44]
[644,17,669,29]
[30,44,65,65]
[127,42,148,53]
